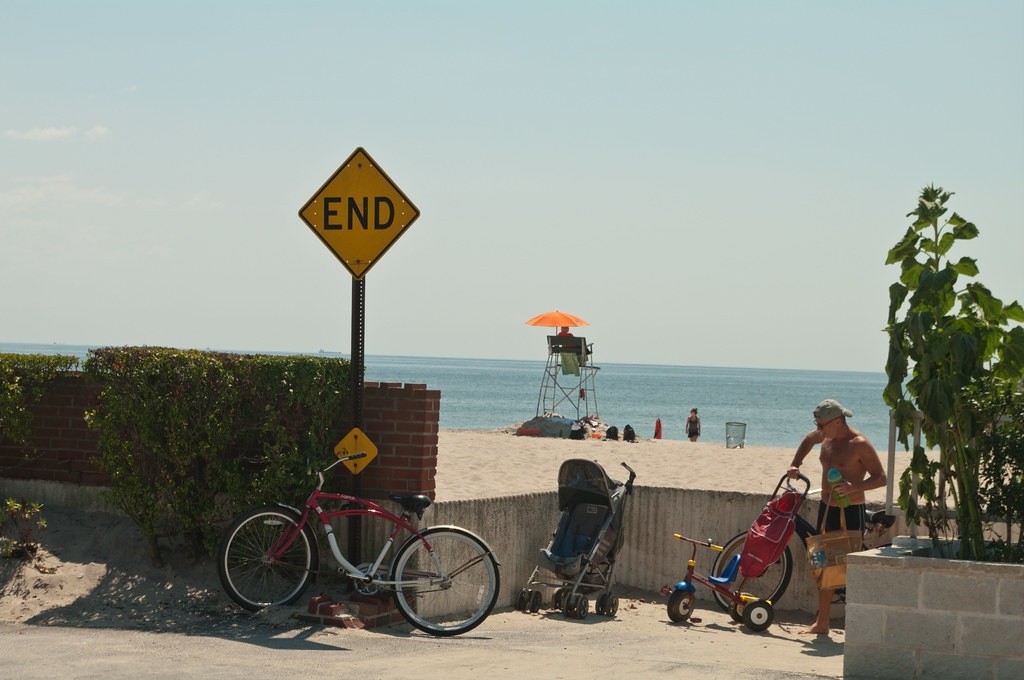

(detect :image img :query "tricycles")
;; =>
[660,473,810,633]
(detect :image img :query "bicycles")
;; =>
[711,473,887,613]
[217,452,500,636]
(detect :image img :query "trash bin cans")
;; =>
[724,422,747,449]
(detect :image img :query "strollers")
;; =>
[516,458,636,619]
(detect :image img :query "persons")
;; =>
[686,408,701,443]
[556,326,593,356]
[787,398,887,634]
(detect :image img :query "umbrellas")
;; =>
[523,310,592,327]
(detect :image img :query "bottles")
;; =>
[826,469,850,509]
